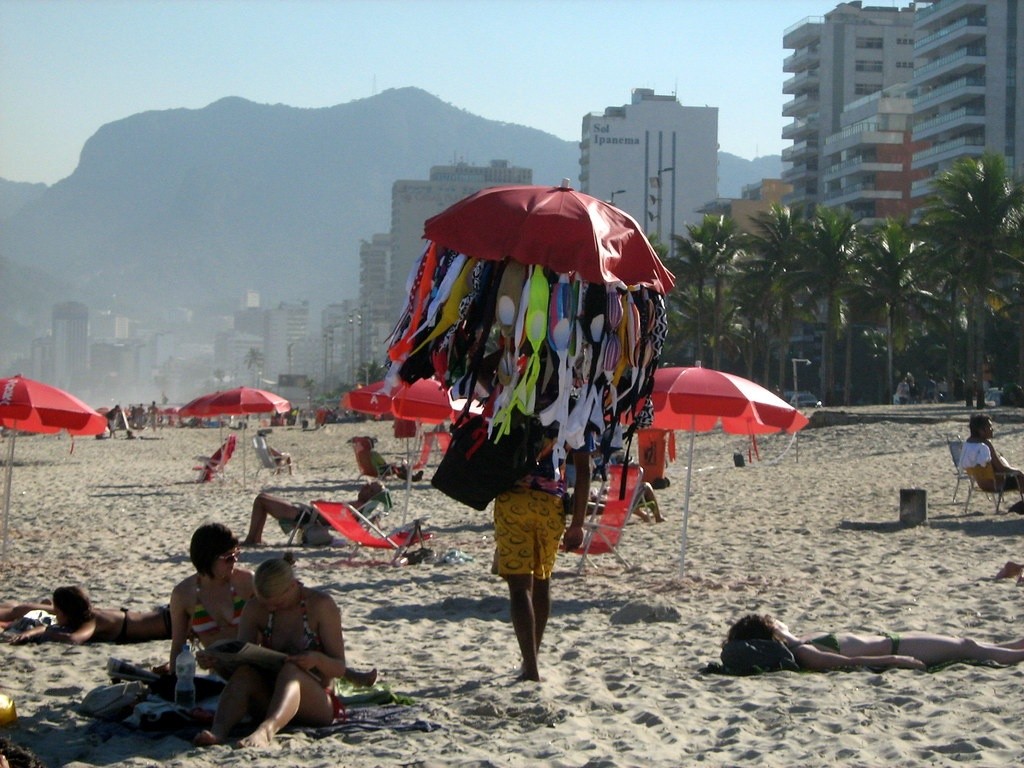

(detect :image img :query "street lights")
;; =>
[792,358,811,395]
[323,310,365,398]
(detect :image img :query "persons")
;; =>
[968,415,1024,502]
[0,523,345,751]
[363,437,425,483]
[490,339,592,685]
[731,613,1024,670]
[259,432,293,476]
[237,481,386,545]
[106,401,357,439]
[895,355,1024,407]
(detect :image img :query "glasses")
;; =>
[218,551,240,564]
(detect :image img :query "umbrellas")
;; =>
[0,374,107,572]
[163,384,290,494]
[345,374,484,527]
[602,359,811,596]
[424,176,675,295]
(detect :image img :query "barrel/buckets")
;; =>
[900,489,925,524]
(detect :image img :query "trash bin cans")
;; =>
[900,490,925,524]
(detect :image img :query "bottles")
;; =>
[175,646,196,711]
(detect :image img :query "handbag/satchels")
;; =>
[431,414,526,511]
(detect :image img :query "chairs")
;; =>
[584,489,659,523]
[351,436,396,484]
[946,433,1023,515]
[251,437,293,481]
[285,489,390,549]
[560,463,643,580]
[307,500,433,567]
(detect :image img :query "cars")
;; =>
[984,387,1004,401]
[790,393,823,409]
